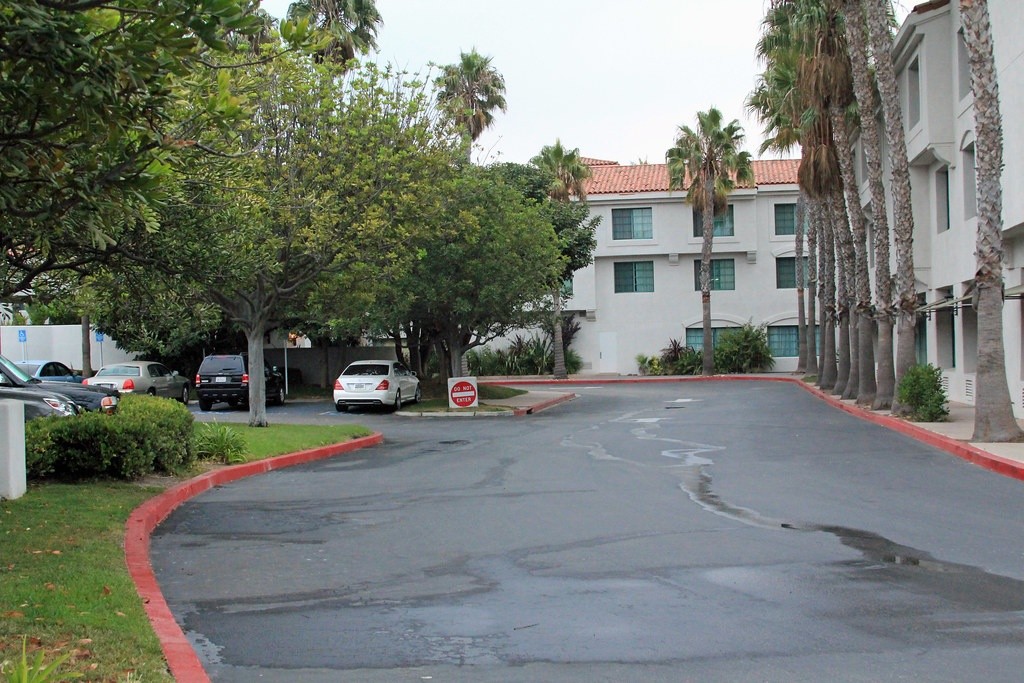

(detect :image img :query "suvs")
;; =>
[195,352,286,410]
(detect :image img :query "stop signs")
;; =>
[448,378,478,407]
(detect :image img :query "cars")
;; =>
[0,354,82,423]
[81,360,190,405]
[333,360,422,413]
[37,380,122,416]
[0,360,87,384]
